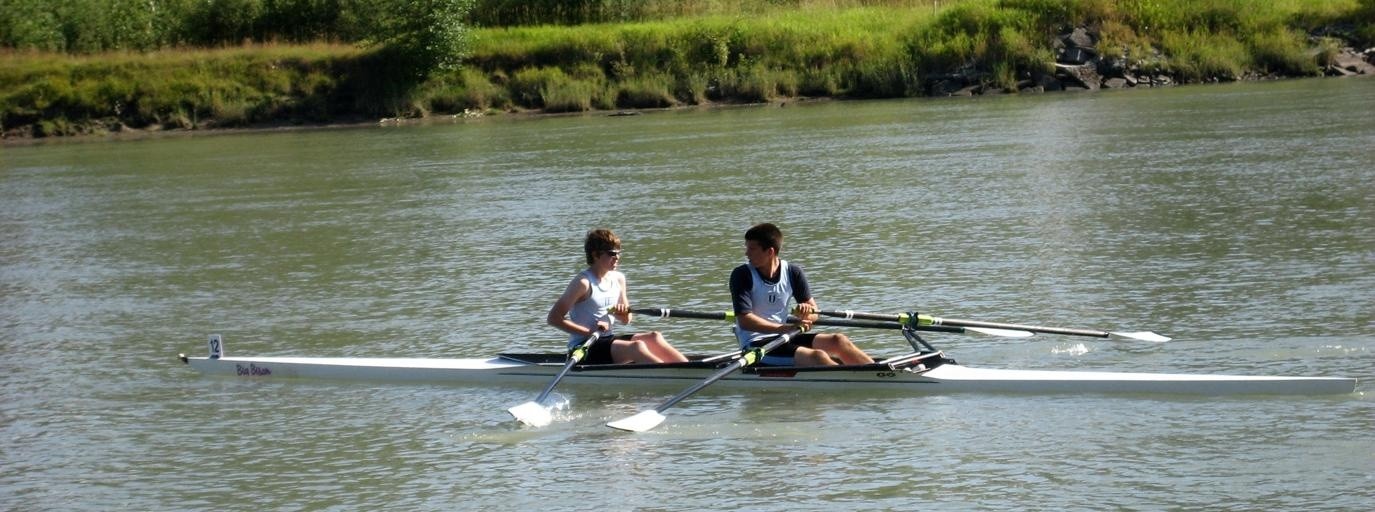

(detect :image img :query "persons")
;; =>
[729,223,876,367]
[547,230,689,365]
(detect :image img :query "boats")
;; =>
[177,350,1357,393]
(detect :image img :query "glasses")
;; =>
[606,251,621,256]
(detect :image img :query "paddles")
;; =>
[792,306,1173,344]
[608,306,1034,339]
[508,326,603,427]
[606,325,807,433]
